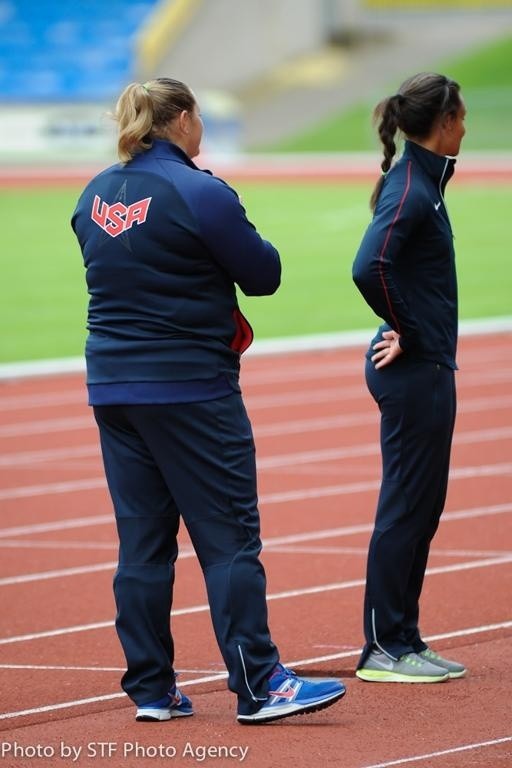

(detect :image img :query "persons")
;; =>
[352,73,467,684]
[70,76,346,727]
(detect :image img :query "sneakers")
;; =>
[416,646,467,679]
[356,644,451,684]
[135,681,194,722]
[235,662,346,723]
